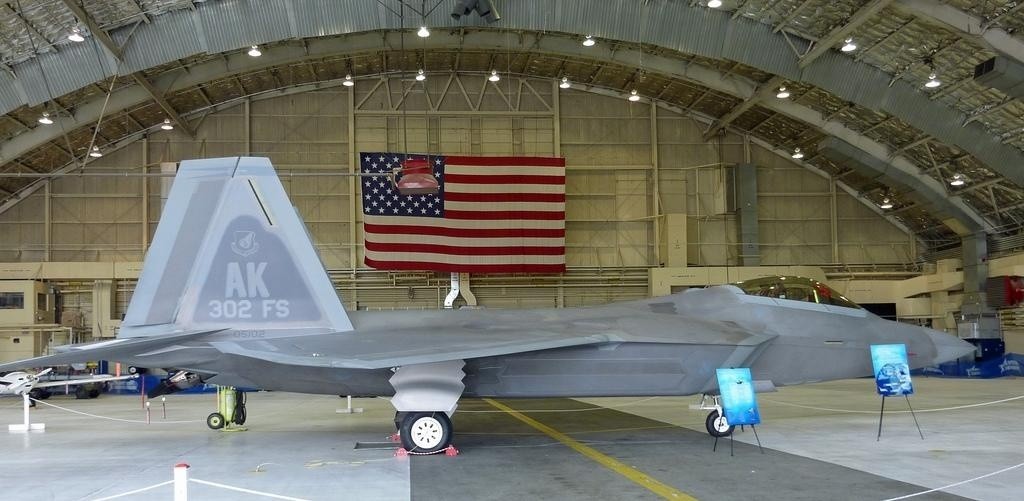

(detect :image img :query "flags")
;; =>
[359,152,566,276]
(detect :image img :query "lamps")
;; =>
[707,0,723,8]
[415,68,426,81]
[842,37,857,52]
[68,24,86,43]
[560,74,569,88]
[247,44,262,57]
[161,113,173,130]
[924,59,941,88]
[777,85,790,98]
[792,147,803,159]
[38,111,54,125]
[582,34,595,47]
[628,88,639,103]
[488,60,501,82]
[880,196,892,208]
[950,172,964,186]
[417,17,430,38]
[343,70,355,87]
[89,135,103,157]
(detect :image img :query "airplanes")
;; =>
[0,366,138,409]
[0,152,976,459]
